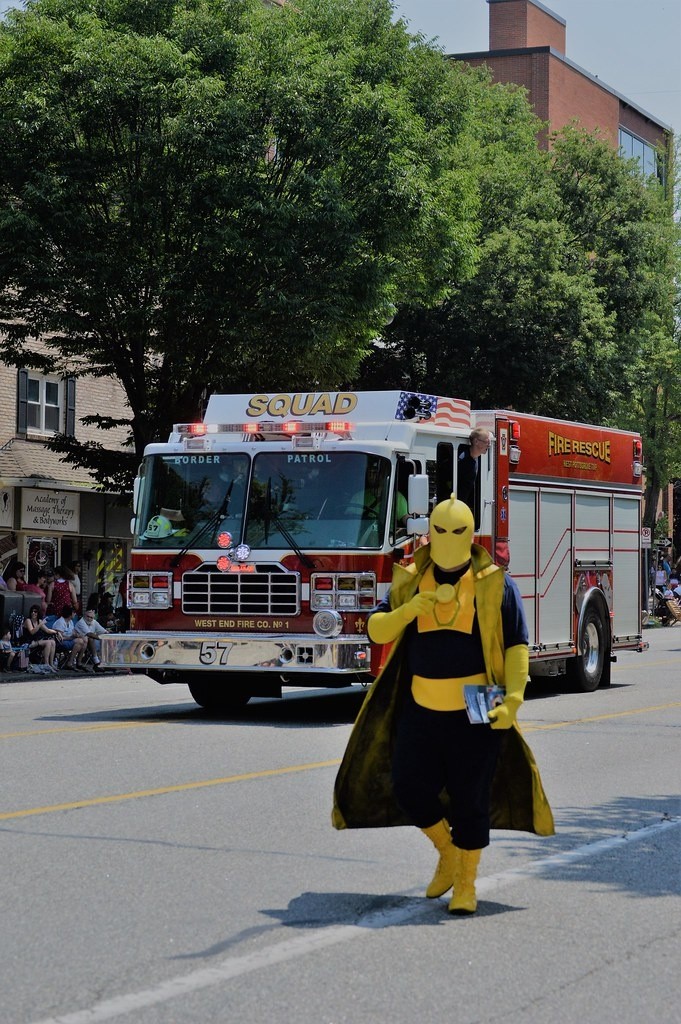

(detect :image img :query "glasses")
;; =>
[658,567,661,568]
[32,611,37,613]
[87,616,92,618]
[20,570,24,572]
[478,439,490,445]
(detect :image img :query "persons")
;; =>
[0,560,127,674]
[155,476,235,522]
[332,492,556,915]
[649,555,681,597]
[345,463,413,533]
[457,428,492,509]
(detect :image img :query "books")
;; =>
[463,684,506,724]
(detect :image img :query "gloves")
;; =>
[368,591,438,644]
[487,644,528,729]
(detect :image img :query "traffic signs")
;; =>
[652,538,673,548]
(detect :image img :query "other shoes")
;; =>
[76,664,88,670]
[50,667,56,673]
[52,666,59,671]
[66,665,78,671]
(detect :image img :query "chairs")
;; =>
[12,616,100,670]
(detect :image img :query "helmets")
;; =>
[429,494,474,571]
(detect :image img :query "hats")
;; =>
[104,592,115,597]
[368,461,385,474]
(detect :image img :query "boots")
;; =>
[421,818,456,899]
[448,847,482,912]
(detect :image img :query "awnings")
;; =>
[0,526,137,542]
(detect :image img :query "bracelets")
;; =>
[156,503,162,515]
[57,631,59,634]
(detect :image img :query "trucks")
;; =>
[97,390,649,706]
